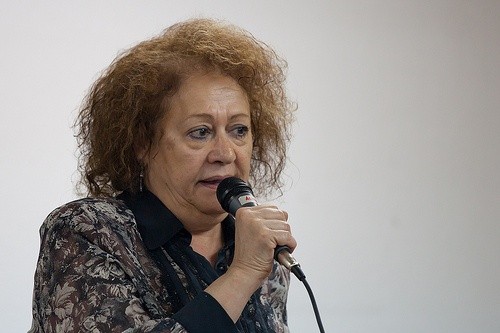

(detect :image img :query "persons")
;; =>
[29,16,306,333]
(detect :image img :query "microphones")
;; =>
[215,177,305,281]
